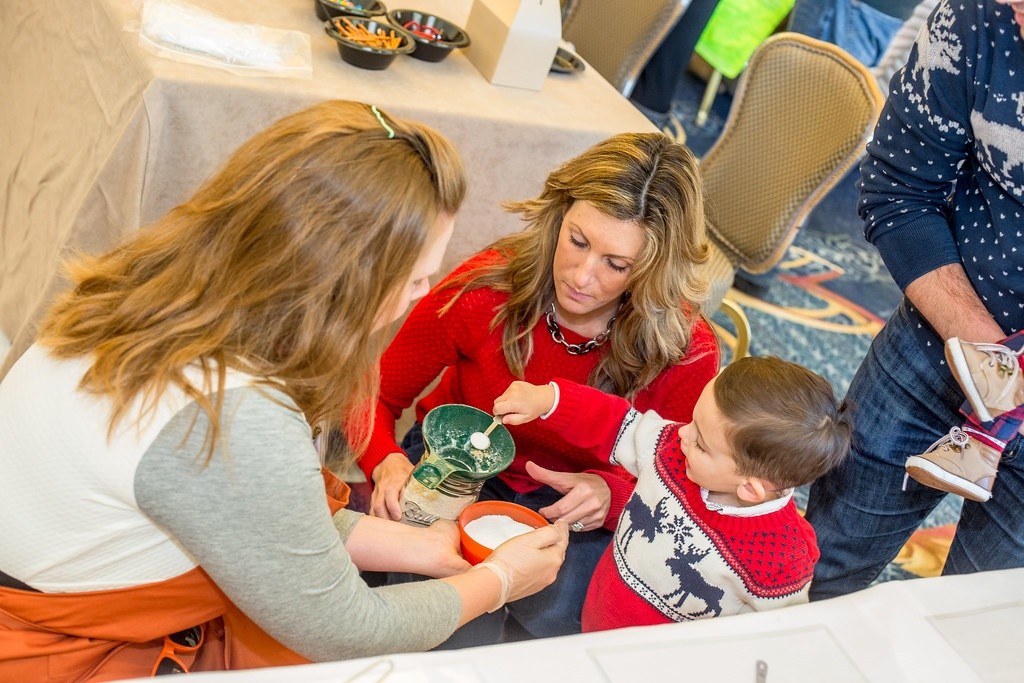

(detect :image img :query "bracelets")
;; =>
[469,563,508,612]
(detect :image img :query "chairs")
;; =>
[558,0,691,99]
[683,31,884,327]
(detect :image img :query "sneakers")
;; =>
[901,424,1001,501]
[945,336,1024,423]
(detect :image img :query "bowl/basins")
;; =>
[386,9,471,62]
[323,16,417,70]
[314,0,387,21]
[458,500,551,567]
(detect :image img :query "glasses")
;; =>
[152,623,203,677]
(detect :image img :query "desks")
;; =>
[1,0,669,389]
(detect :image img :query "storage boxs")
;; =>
[461,0,562,93]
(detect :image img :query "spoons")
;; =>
[470,416,502,451]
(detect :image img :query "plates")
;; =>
[550,47,585,73]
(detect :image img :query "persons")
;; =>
[804,0,1024,602]
[630,0,924,120]
[342,134,721,652]
[492,355,851,635]
[0,99,568,683]
[904,330,1024,502]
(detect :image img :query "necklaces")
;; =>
[544,299,625,355]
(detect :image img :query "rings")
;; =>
[571,521,584,532]
